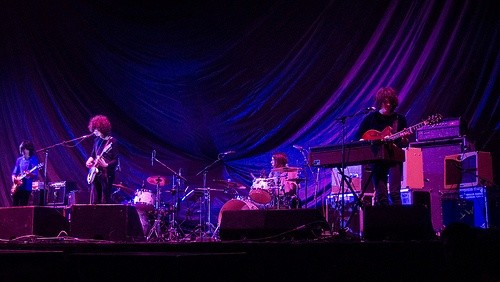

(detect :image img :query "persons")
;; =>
[86,115,118,204]
[355,86,414,206]
[268,153,301,208]
[12,140,39,206]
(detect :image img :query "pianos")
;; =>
[309,140,406,234]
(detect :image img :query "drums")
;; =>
[249,178,275,204]
[134,189,155,211]
[217,197,259,228]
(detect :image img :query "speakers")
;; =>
[45,185,67,204]
[218,210,328,242]
[444,151,494,189]
[66,204,150,240]
[357,207,438,242]
[406,145,461,233]
[441,191,500,230]
[67,190,90,205]
[29,189,44,205]
[331,163,377,192]
[0,207,63,239]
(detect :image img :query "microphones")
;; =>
[89,130,100,137]
[223,151,235,155]
[151,151,155,166]
[363,107,376,113]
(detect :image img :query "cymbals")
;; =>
[147,175,171,186]
[112,184,133,192]
[213,181,246,189]
[195,187,224,191]
[286,178,309,181]
[271,166,302,171]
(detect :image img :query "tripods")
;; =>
[147,156,228,240]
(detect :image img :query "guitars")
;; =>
[384,115,442,143]
[87,143,112,185]
[8,163,44,198]
